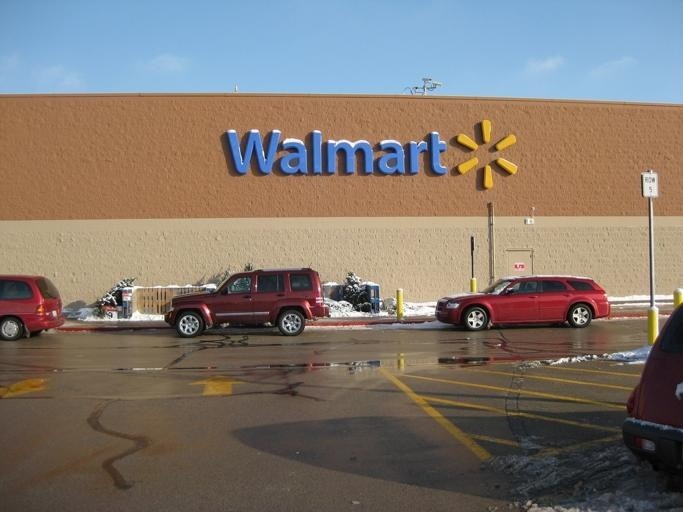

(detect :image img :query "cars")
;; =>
[434,275,609,331]
[617,301,683,474]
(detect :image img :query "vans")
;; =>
[0,274,64,340]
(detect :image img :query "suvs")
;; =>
[165,268,330,337]
